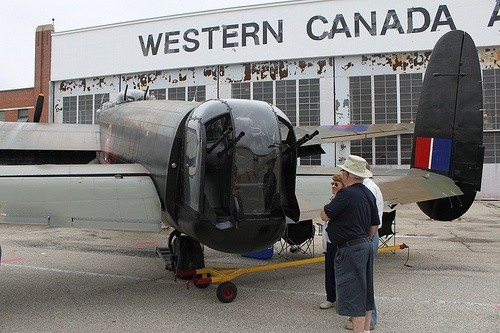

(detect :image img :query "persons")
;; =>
[318,154,384,333]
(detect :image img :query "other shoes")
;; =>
[319,300,335,309]
[346,317,375,330]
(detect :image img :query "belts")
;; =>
[338,239,370,249]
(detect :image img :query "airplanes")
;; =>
[0,29,486,279]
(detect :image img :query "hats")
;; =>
[336,153,372,178]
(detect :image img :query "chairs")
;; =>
[378,209,398,254]
[278,219,315,259]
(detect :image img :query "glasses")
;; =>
[331,182,343,187]
[340,169,346,173]
